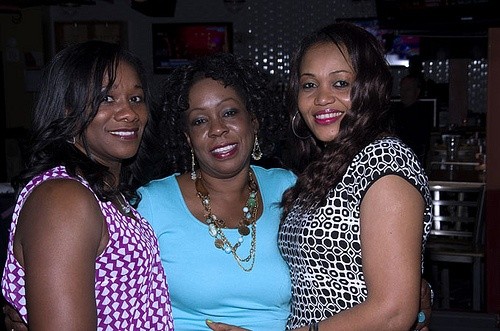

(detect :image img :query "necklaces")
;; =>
[195,174,259,272]
[101,181,131,213]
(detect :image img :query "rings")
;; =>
[418,312,427,324]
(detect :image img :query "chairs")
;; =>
[429,185,487,312]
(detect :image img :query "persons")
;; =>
[2,56,434,331]
[1,41,174,331]
[206,22,434,331]
[388,75,431,143]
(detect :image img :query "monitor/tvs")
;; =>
[150,21,234,76]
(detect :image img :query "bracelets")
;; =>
[424,279,434,309]
[309,322,319,331]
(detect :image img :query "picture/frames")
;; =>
[153,21,234,74]
